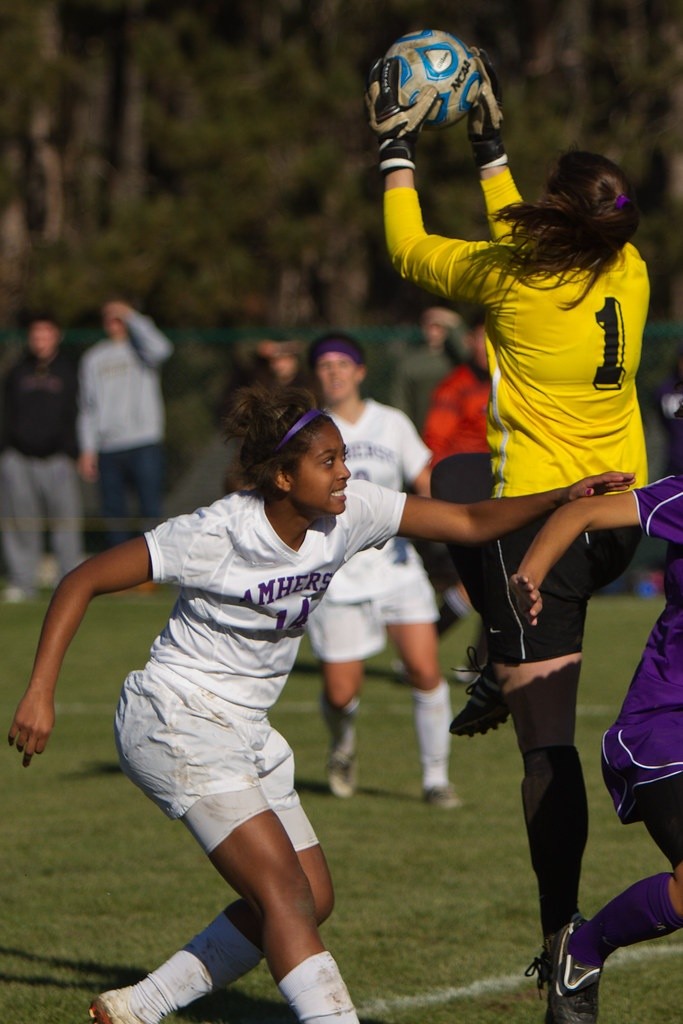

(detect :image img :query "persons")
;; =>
[220,300,501,685]
[7,383,635,1024]
[506,468,682,1023]
[297,337,466,814]
[1,322,93,603]
[356,52,652,994]
[75,297,173,596]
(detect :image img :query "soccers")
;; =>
[383,28,480,130]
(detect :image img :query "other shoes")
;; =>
[453,655,476,678]
[327,725,359,800]
[425,783,462,811]
[5,585,31,604]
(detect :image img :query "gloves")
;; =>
[464,47,507,168]
[364,57,437,170]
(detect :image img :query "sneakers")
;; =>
[88,985,146,1024]
[524,929,557,998]
[546,918,603,1024]
[449,645,510,737]
